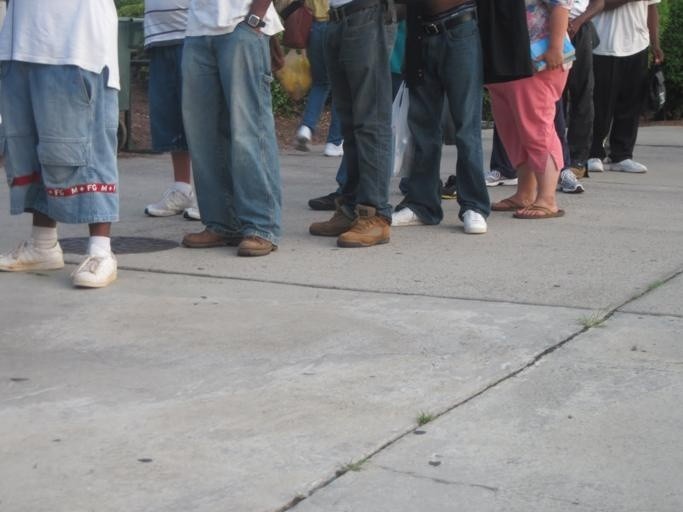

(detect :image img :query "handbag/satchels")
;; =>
[281,5,315,51]
[646,62,668,112]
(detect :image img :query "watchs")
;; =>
[244,14,266,28]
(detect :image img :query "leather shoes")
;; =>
[182,227,240,248]
[237,236,278,256]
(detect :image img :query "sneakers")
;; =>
[294,125,315,154]
[71,249,118,288]
[0,237,65,272]
[324,140,344,158]
[145,188,202,220]
[484,158,648,219]
[308,171,485,247]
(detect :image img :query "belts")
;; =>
[328,0,382,22]
[416,10,477,37]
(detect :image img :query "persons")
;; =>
[182,0,283,256]
[0,0,121,288]
[143,0,200,221]
